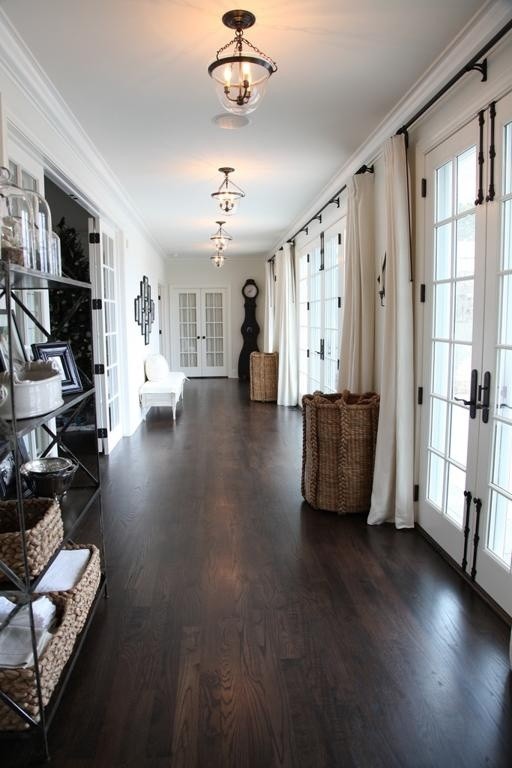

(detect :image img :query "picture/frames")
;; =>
[32,341,83,395]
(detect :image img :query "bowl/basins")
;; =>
[23,457,80,499]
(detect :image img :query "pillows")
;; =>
[146,356,170,382]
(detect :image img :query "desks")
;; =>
[140,372,185,420]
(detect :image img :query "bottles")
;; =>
[1,167,63,277]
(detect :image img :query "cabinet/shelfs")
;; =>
[0,260,109,760]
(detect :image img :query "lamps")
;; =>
[209,250,229,268]
[207,9,278,116]
[210,167,245,216]
[210,220,233,253]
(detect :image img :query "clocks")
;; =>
[244,284,257,298]
